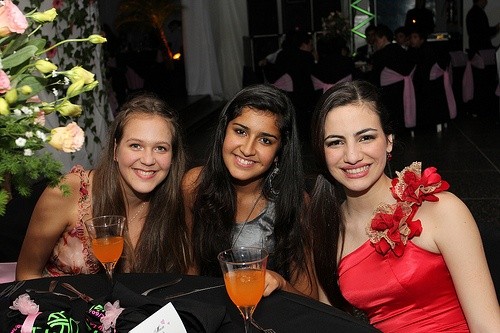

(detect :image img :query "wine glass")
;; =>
[85,214,127,307]
[218,247,270,333]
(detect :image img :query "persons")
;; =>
[181,85,318,300]
[101,10,182,100]
[259,0,434,80]
[16,97,188,281]
[466,0,500,51]
[310,81,500,333]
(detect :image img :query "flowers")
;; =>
[0,0,109,217]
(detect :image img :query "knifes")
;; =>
[63,283,94,303]
[165,285,224,300]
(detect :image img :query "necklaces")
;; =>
[128,201,146,225]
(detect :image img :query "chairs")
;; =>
[260,47,500,138]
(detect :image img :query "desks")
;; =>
[0,272,382,333]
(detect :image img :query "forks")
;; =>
[250,318,277,333]
